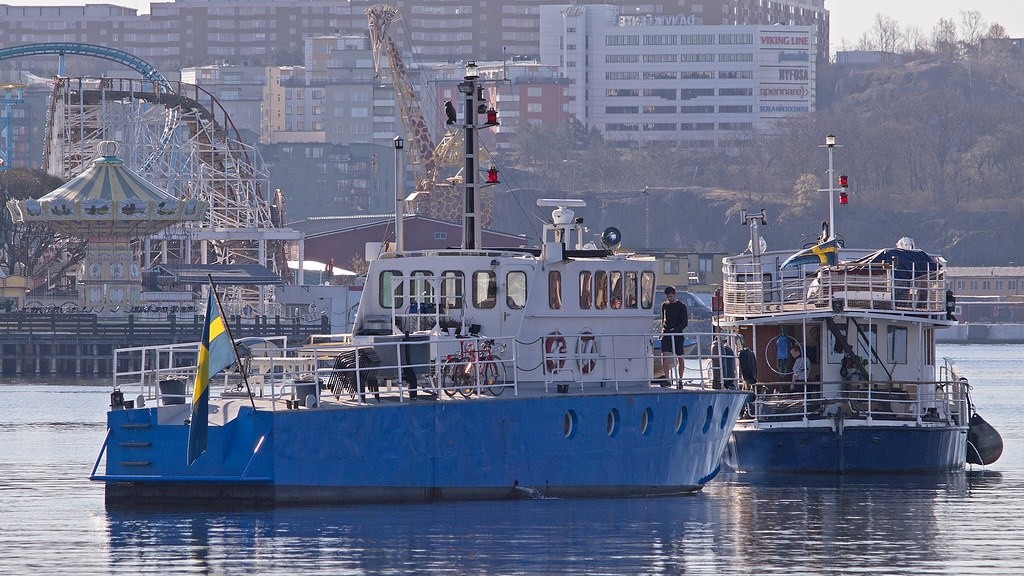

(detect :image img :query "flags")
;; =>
[187,288,236,466]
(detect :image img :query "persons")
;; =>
[789,345,811,395]
[657,285,688,391]
[711,336,735,390]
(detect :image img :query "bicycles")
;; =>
[441,332,507,398]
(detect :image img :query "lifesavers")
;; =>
[544,332,567,373]
[738,348,759,385]
[576,331,600,374]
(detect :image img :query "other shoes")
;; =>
[679,381,683,389]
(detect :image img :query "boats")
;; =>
[84,136,758,507]
[701,133,1004,472]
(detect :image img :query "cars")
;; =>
[653,290,715,319]
